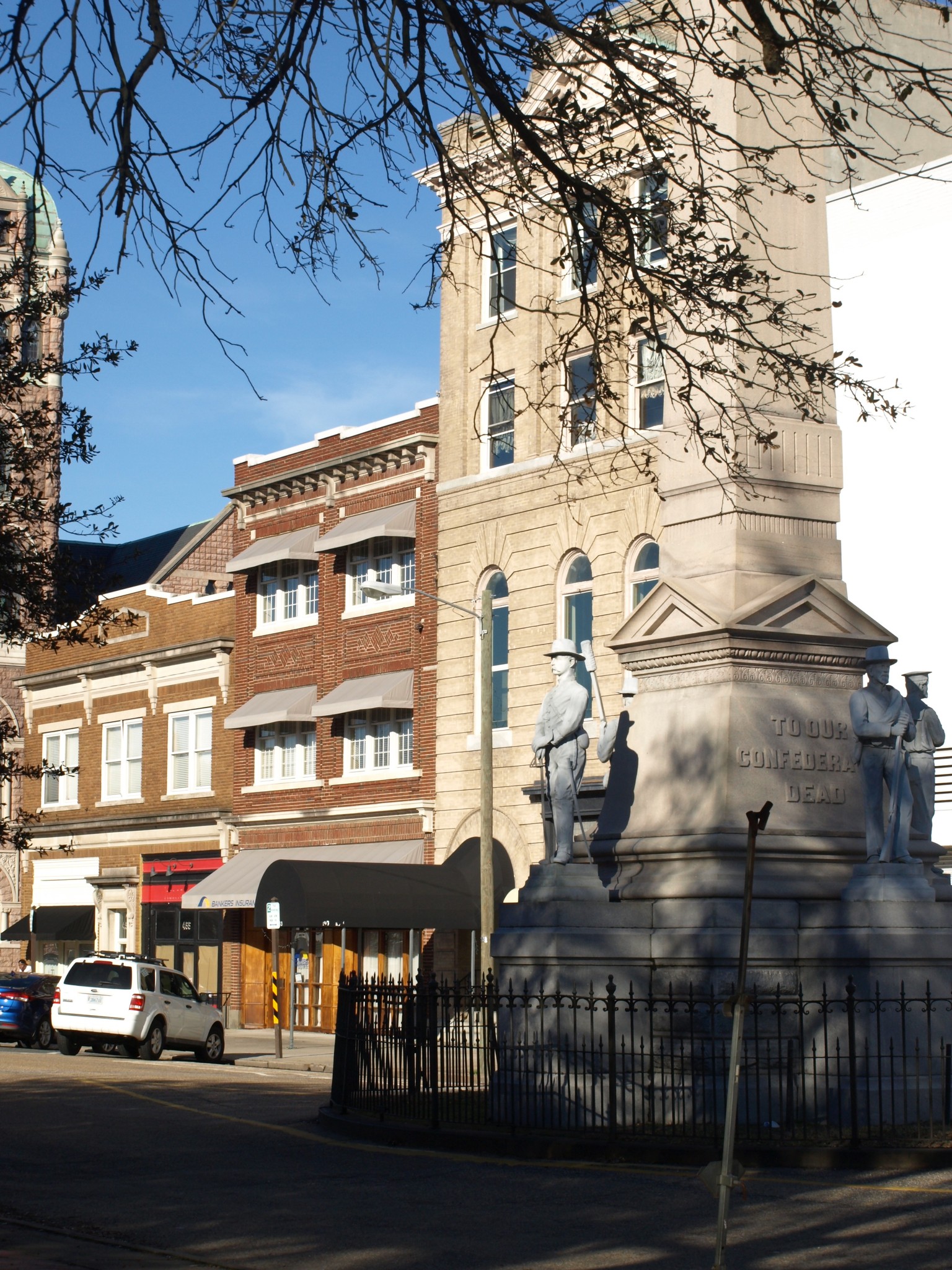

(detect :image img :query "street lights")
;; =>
[362,580,495,1090]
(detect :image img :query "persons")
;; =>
[848,644,922,864]
[902,669,945,874]
[18,959,32,974]
[596,665,640,789]
[530,638,590,866]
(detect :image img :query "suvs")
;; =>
[49,951,230,1061]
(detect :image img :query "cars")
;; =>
[0,973,65,1049]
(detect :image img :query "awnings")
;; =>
[312,668,414,716]
[223,683,316,732]
[178,838,487,930]
[0,904,95,942]
[226,525,320,575]
[314,500,416,553]
